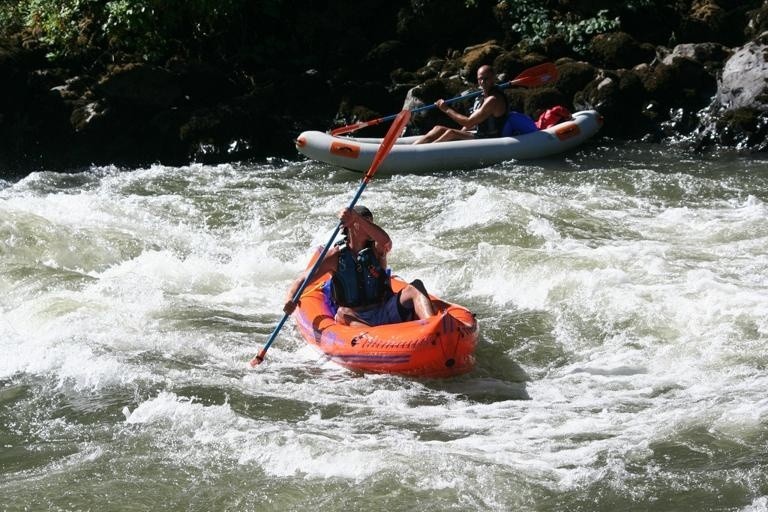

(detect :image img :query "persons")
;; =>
[281,205,436,328]
[408,64,509,145]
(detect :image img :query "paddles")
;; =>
[249,110,411,366]
[330,63,560,138]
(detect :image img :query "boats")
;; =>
[295,109,600,177]
[294,245,478,380]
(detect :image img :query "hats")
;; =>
[340,205,374,235]
